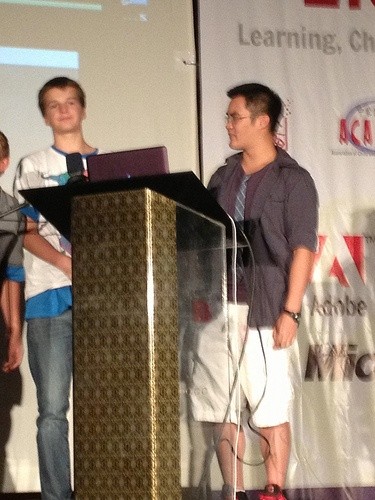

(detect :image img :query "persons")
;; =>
[187,83,319,500]
[13,77,114,500]
[0,130,27,374]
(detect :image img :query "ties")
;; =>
[233,174,252,283]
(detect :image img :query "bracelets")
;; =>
[281,306,302,329]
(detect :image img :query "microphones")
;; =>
[66,152,84,185]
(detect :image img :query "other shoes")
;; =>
[258,484,285,500]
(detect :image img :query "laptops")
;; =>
[86,146,169,182]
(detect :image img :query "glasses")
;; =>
[225,114,259,124]
